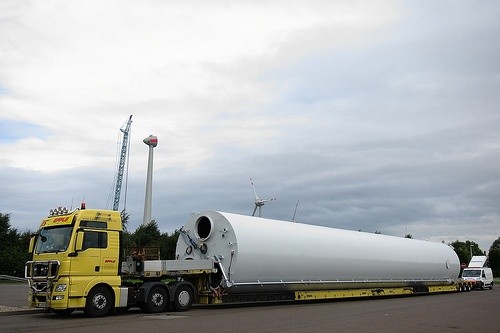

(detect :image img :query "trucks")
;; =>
[25,203,476,318]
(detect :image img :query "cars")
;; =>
[461,262,467,267]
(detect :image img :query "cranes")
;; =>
[104,115,133,211]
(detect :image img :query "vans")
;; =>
[461,268,494,290]
